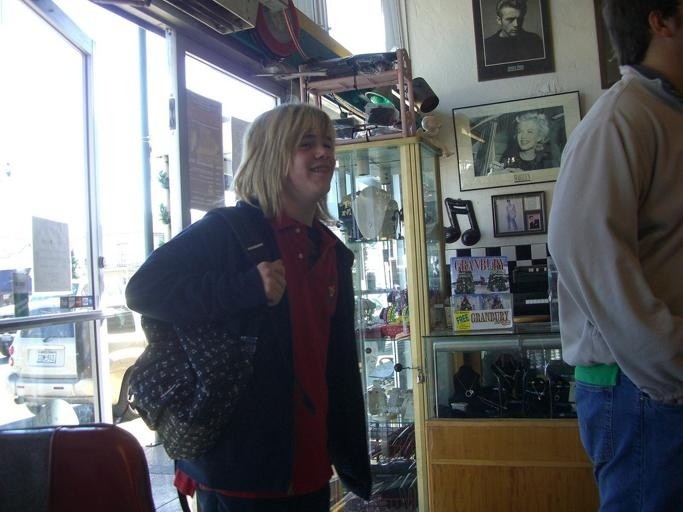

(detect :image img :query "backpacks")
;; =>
[127,207,274,460]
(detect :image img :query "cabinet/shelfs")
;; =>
[320,135,450,510]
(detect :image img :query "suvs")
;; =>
[8,303,149,423]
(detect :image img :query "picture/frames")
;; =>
[451,90,582,190]
[491,192,548,238]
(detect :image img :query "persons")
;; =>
[506,198,518,232]
[484,1,544,66]
[124,103,372,512]
[547,1,682,510]
[501,113,561,171]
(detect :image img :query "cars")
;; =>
[0,305,77,359]
